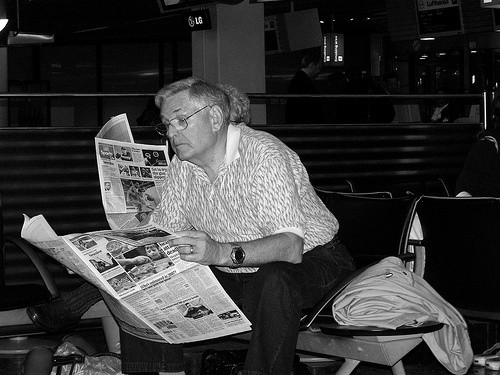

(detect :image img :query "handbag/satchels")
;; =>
[50,342,125,375]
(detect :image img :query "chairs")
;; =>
[180,128,500,375]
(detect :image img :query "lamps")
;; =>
[7,0,56,46]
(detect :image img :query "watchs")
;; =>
[231,243,245,270]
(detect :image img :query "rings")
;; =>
[189,245,193,254]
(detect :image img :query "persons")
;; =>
[116,77,353,374]
[115,244,167,267]
[27,83,251,331]
[286,52,498,124]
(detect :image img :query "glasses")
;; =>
[155,105,212,136]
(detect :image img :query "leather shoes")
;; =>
[26,297,80,333]
